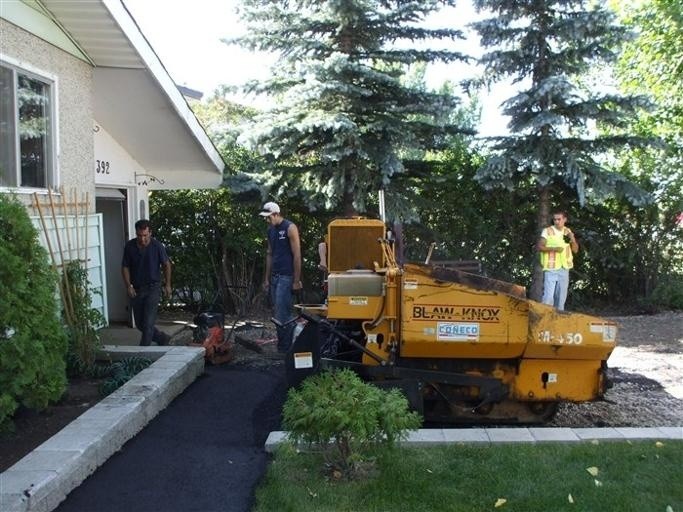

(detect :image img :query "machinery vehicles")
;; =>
[270,185,620,426]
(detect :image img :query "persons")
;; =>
[538,211,578,311]
[258,202,301,359]
[122,220,171,345]
[318,243,327,271]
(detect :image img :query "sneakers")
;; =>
[159,332,172,346]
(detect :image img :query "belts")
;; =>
[272,271,293,277]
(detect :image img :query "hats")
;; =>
[257,200,282,219]
